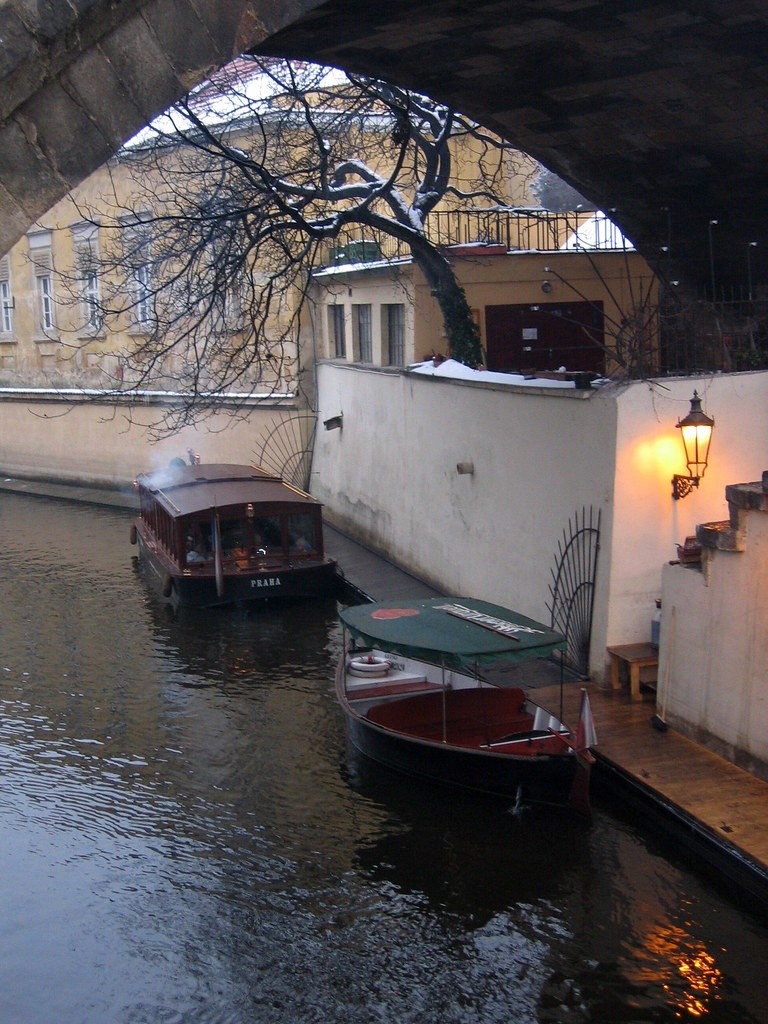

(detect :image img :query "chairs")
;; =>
[606,641,659,702]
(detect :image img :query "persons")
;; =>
[187,540,206,561]
[241,524,261,547]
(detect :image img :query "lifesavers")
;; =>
[349,654,392,677]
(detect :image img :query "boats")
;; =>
[334,595,598,828]
[131,447,339,613]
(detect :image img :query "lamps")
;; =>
[671,389,714,500]
[323,415,343,430]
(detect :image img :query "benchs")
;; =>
[458,730,570,754]
[366,687,534,749]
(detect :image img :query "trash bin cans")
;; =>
[329,240,382,266]
